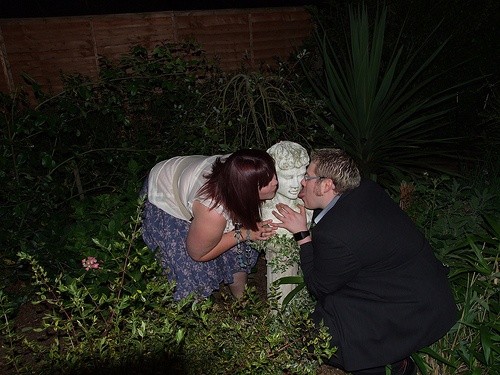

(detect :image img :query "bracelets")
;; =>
[234,228,252,248]
[293,231,310,241]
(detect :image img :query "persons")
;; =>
[259,141,315,238]
[143,150,280,310]
[269,148,459,375]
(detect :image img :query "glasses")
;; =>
[304,175,327,181]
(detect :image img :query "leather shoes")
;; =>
[395,358,417,375]
